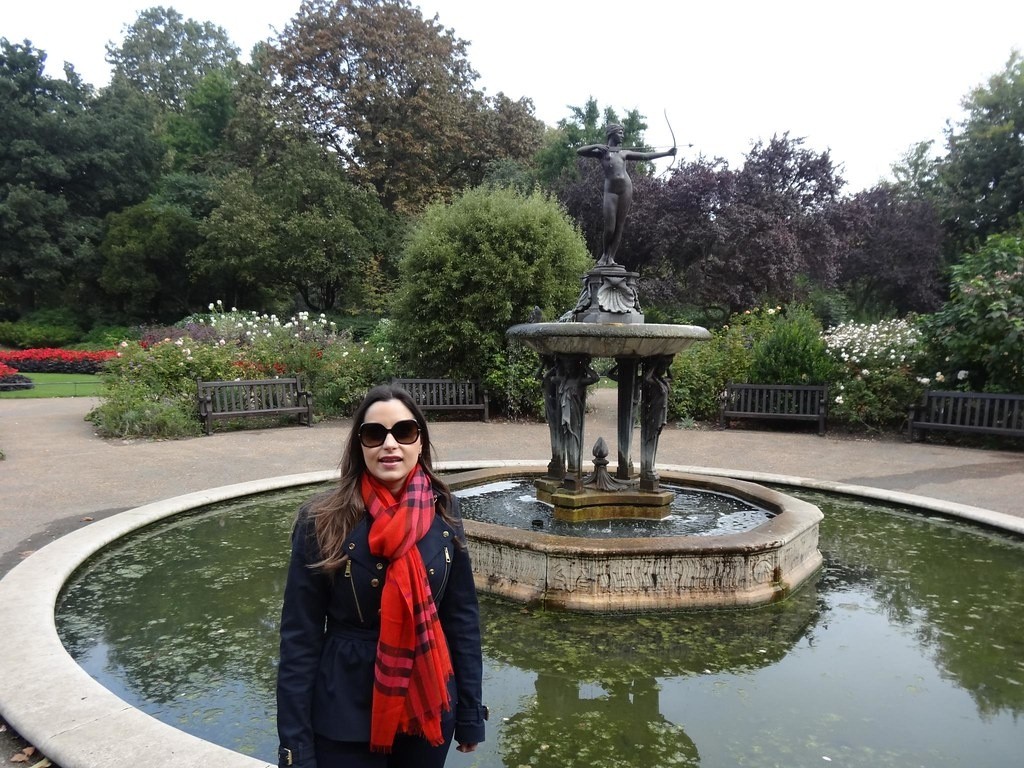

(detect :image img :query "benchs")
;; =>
[718,380,828,436]
[392,374,491,421]
[906,389,1024,443]
[195,375,315,436]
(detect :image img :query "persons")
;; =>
[577,124,677,266]
[537,354,599,471]
[607,360,672,474]
[276,386,489,768]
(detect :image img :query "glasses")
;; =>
[358,419,421,448]
